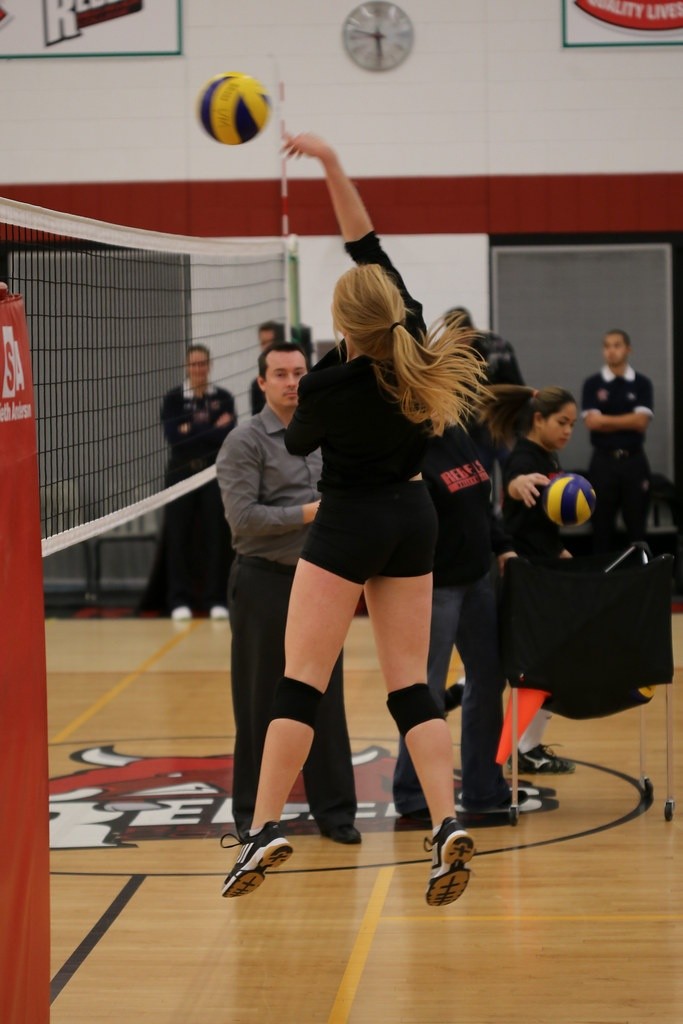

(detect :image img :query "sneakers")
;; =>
[220,820,294,901]
[507,743,575,774]
[423,817,476,907]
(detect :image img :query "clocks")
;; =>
[341,1,415,72]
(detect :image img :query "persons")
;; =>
[148,344,237,621]
[473,384,578,578]
[388,354,515,834]
[581,330,653,537]
[445,309,527,441]
[217,340,362,845]
[249,322,313,418]
[220,134,486,910]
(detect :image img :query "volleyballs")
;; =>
[200,71,273,144]
[542,473,597,528]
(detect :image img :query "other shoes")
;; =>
[210,606,229,620]
[394,800,432,816]
[236,829,250,840]
[461,790,528,811]
[319,825,361,845]
[172,606,191,620]
